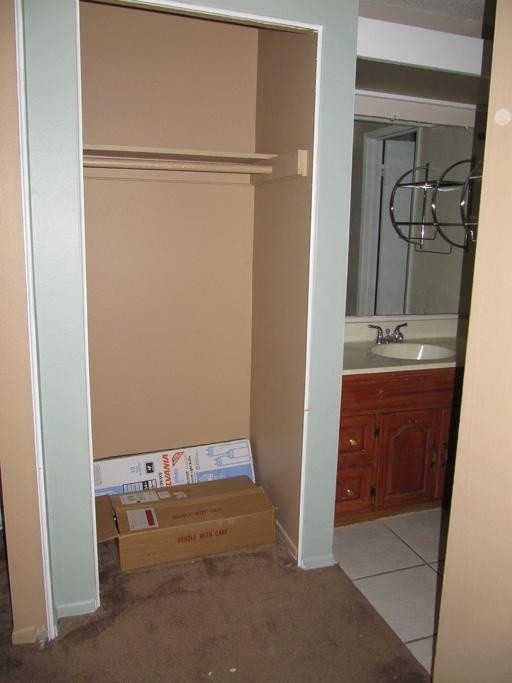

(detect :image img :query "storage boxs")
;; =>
[94,475,277,572]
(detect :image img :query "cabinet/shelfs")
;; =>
[333,366,455,529]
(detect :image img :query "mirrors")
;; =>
[345,88,477,323]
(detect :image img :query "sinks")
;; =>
[371,343,458,360]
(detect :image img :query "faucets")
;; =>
[384,329,398,344]
